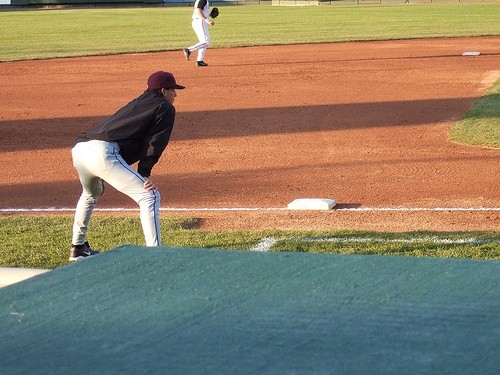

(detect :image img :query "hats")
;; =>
[147,71,186,90]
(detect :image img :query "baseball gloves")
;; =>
[210,7,220,19]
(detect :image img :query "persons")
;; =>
[69,71,187,262]
[183,0,219,66]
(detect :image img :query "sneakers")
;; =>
[183,48,191,60]
[68,240,102,261]
[197,61,208,66]
